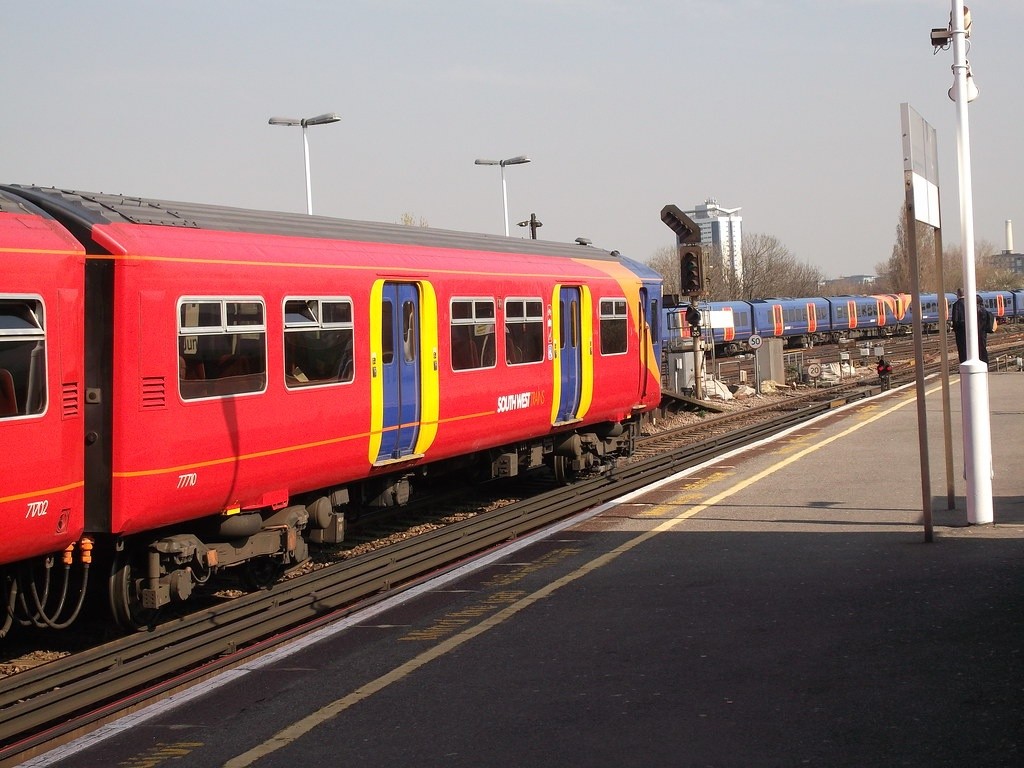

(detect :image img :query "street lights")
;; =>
[268,113,342,212]
[474,156,532,238]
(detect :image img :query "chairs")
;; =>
[483,337,517,367]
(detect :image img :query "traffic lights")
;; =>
[877,362,892,375]
[685,305,701,324]
[680,245,702,296]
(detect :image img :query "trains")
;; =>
[662,288,1024,358]
[0,187,668,644]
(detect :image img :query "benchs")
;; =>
[186,356,209,397]
[453,340,481,368]
[210,352,250,395]
[179,352,187,395]
[0,367,19,417]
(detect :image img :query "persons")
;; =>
[952,288,967,375]
[977,295,988,368]
[183,347,207,397]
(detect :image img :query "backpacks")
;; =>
[981,306,998,333]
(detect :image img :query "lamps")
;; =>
[946,55,980,103]
[929,5,974,49]
